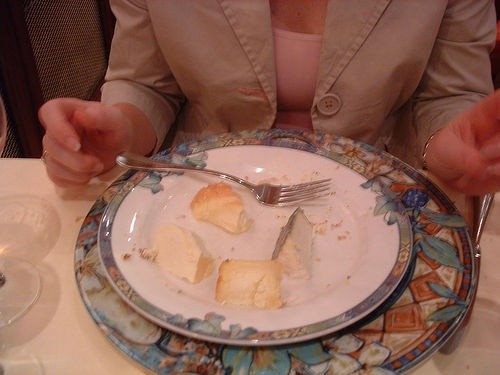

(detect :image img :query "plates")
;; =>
[97,137,414,346]
[74,128,475,375]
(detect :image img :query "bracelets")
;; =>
[421,131,442,169]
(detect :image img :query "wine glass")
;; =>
[0,97,41,328]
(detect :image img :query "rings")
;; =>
[41,150,49,163]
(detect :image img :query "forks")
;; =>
[116,151,333,207]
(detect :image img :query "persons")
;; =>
[39,0,500,196]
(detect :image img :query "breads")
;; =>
[271,205,314,280]
[214,258,282,309]
[155,225,215,282]
[191,180,249,233]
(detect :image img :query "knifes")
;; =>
[438,193,494,355]
